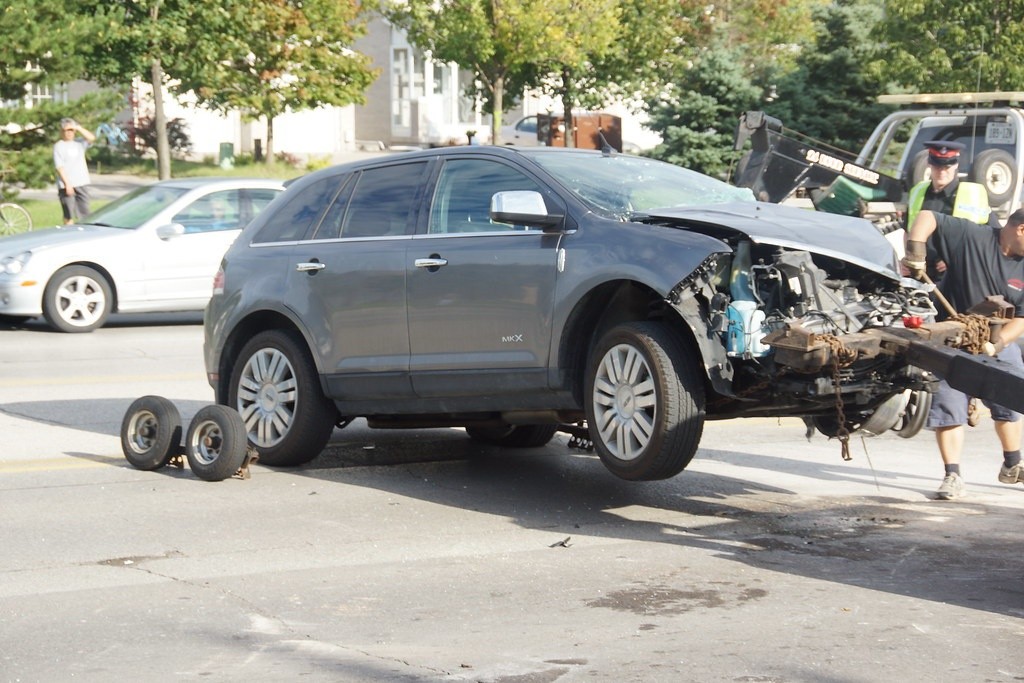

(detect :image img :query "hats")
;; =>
[924,141,966,166]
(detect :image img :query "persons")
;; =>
[53,118,95,226]
[902,208,1024,500]
[903,141,1002,283]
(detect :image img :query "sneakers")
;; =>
[998,460,1024,484]
[936,472,964,499]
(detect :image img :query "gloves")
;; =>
[982,336,1004,358]
[901,240,927,281]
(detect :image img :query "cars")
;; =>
[203,146,940,482]
[0,178,296,333]
[487,114,546,149]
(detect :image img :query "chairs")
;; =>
[452,173,515,232]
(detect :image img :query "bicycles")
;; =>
[0,174,34,238]
